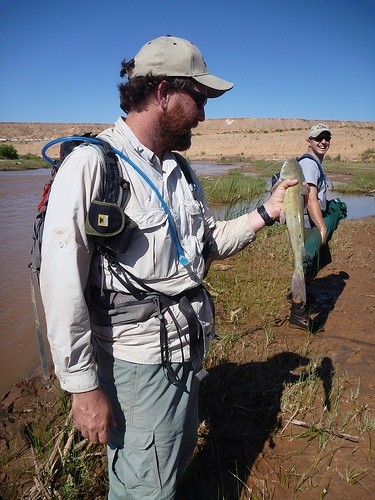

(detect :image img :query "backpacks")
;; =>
[272,154,323,224]
[28,132,198,380]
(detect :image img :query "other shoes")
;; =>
[287,292,315,302]
[289,314,323,332]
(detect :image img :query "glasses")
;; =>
[313,136,332,142]
[187,88,207,110]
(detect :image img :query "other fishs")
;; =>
[266,160,309,304]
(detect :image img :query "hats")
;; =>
[128,37,234,98]
[308,124,331,139]
